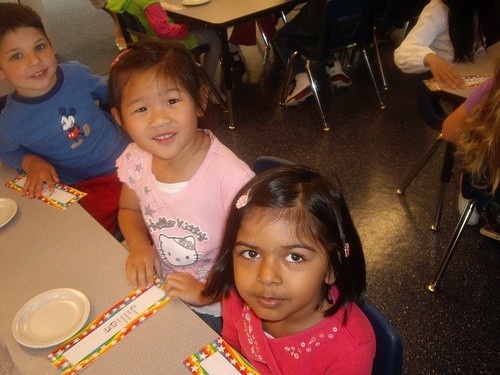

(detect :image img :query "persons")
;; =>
[203,164,376,375]
[103,0,228,105]
[0,2,134,237]
[439,57,500,241]
[272,1,352,106]
[392,0,500,225]
[226,14,279,72]
[109,40,257,337]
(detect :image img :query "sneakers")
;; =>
[285,72,318,106]
[458,174,480,225]
[325,61,352,87]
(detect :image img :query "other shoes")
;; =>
[208,92,227,104]
[229,47,246,74]
[347,47,369,68]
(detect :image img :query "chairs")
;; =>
[397,16,458,231]
[427,169,500,292]
[256,0,428,131]
[100,4,227,112]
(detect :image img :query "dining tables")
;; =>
[0,161,221,375]
[432,42,500,104]
[164,0,286,95]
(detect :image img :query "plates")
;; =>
[12,287,90,349]
[0,197,17,228]
[181,0,210,6]
